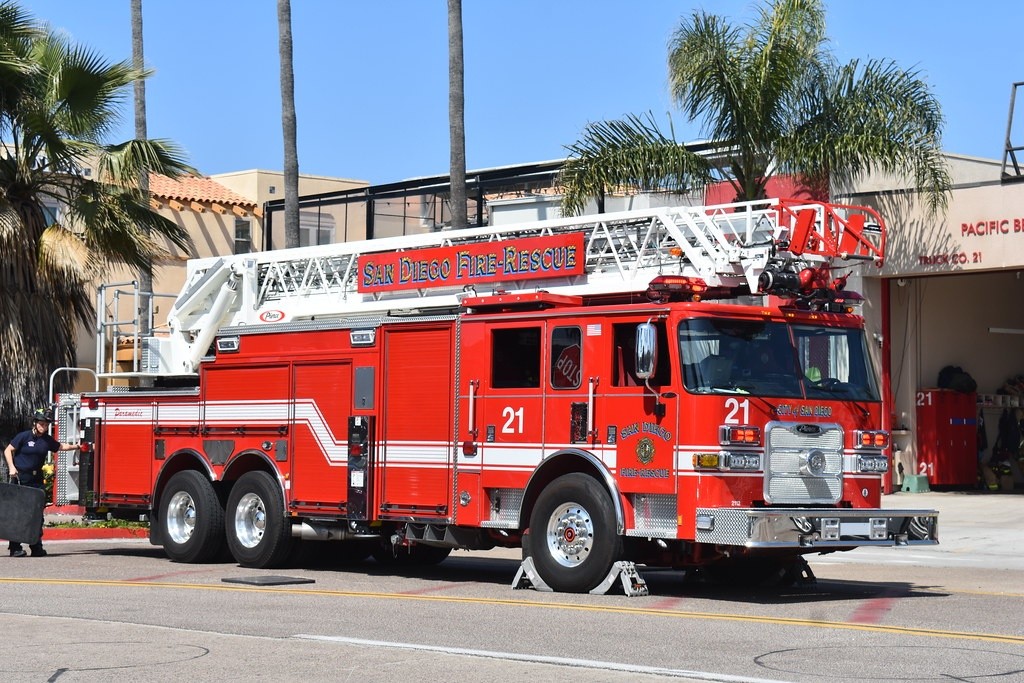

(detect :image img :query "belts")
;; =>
[20,469,44,476]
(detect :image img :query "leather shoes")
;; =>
[31,549,47,557]
[7,547,27,556]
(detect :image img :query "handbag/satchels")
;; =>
[0,475,46,545]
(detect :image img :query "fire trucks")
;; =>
[45,195,942,596]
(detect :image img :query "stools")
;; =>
[901,474,930,493]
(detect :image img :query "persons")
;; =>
[4,409,84,556]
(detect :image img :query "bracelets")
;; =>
[76,444,80,451]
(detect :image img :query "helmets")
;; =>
[32,408,54,423]
